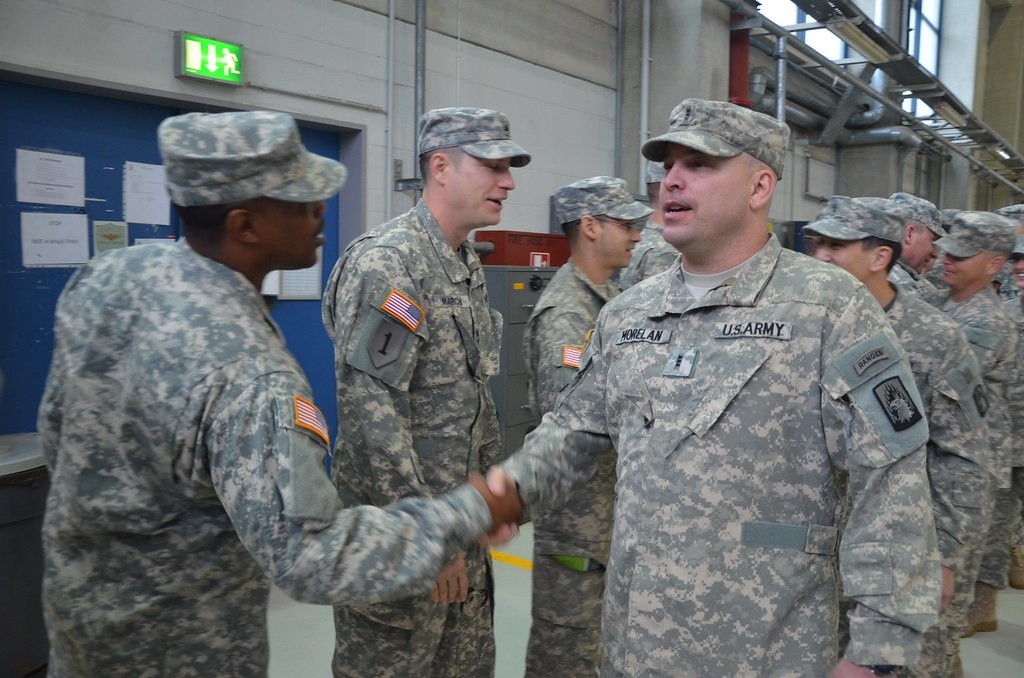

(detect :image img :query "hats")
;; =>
[799,196,908,245]
[931,203,1024,258]
[418,108,531,168]
[642,97,790,182]
[887,193,947,236]
[555,176,655,220]
[157,112,347,206]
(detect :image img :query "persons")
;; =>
[477,98,941,678]
[518,176,655,678]
[321,105,532,678]
[802,191,1024,678]
[37,110,522,678]
[619,162,680,293]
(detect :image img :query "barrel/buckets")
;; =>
[0,431,54,678]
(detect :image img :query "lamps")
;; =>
[825,12,889,65]
[933,102,967,127]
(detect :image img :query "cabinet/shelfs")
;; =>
[477,266,561,525]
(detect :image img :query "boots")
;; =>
[963,581,997,639]
[1008,544,1024,588]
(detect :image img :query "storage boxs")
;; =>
[475,230,571,267]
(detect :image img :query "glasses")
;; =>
[594,218,641,230]
